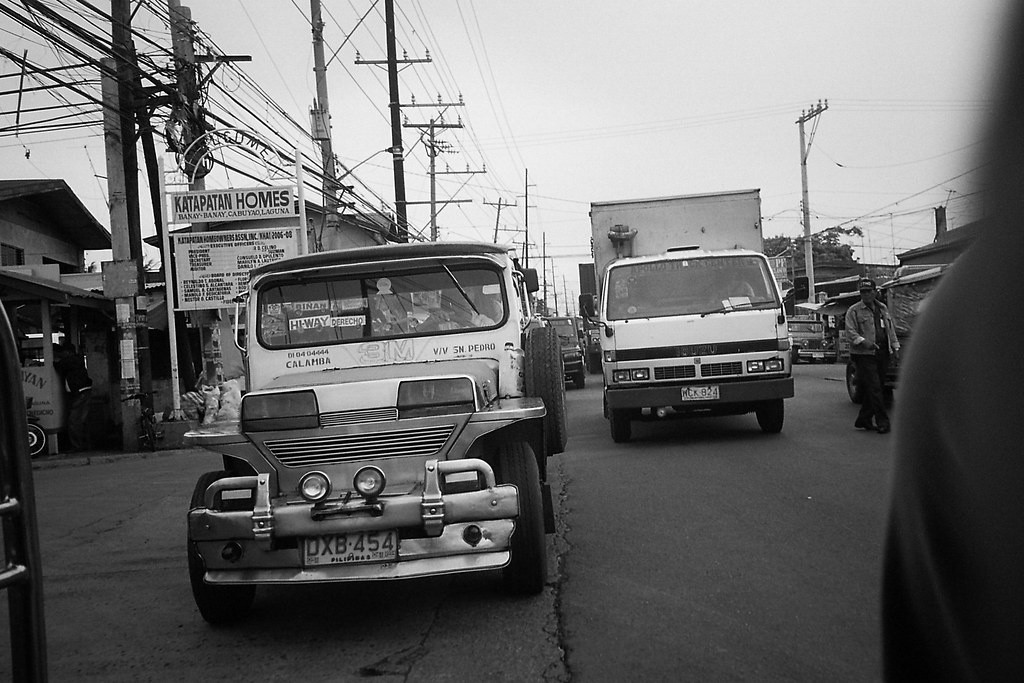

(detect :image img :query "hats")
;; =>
[858,279,877,291]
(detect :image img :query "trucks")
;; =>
[844,261,957,405]
[589,186,810,446]
[543,313,601,392]
[785,313,841,365]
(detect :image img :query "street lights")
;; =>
[325,143,404,251]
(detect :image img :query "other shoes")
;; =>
[855,420,878,430]
[878,424,890,433]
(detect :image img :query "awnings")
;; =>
[794,302,858,316]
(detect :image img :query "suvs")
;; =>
[183,242,572,628]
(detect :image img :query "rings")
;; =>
[439,313,444,317]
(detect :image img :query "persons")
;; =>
[708,271,755,305]
[881,0,1024,683]
[845,279,900,433]
[53,343,94,453]
[416,287,495,332]
[821,314,830,331]
